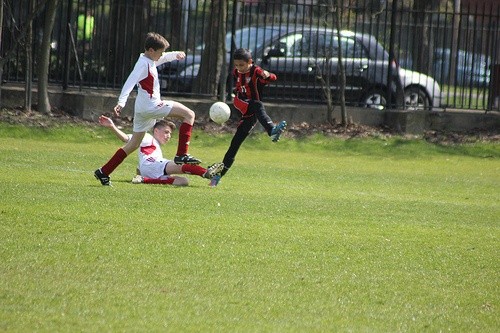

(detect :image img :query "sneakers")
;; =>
[270,120,287,142]
[174,154,201,166]
[203,163,224,178]
[93,167,111,185]
[132,175,143,183]
[208,172,222,187]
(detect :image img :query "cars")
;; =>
[136,23,401,110]
[403,47,492,87]
[398,67,444,111]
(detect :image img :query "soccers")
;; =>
[209,101,231,124]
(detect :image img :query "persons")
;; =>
[94,32,202,186]
[209,48,286,187]
[98,115,189,187]
